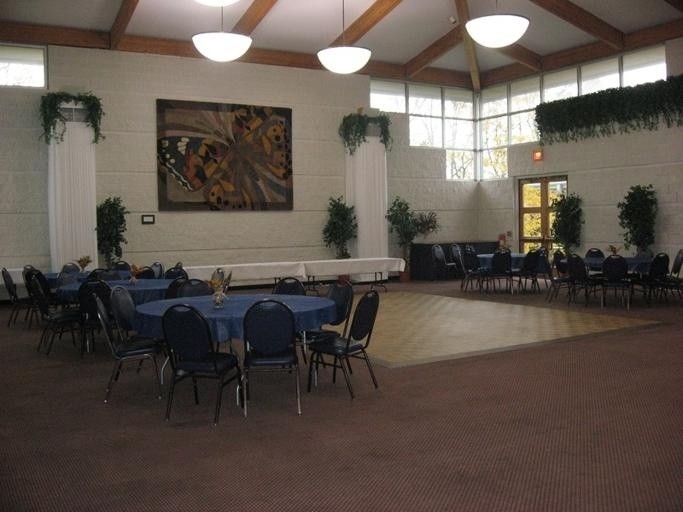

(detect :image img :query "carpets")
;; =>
[313,289,663,370]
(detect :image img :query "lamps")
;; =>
[191,7,252,64]
[314,1,372,75]
[463,1,530,49]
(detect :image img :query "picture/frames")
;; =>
[155,98,293,211]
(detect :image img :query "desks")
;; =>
[184,258,305,289]
[304,257,407,294]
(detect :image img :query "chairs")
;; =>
[429,241,682,313]
[110,278,356,375]
[89,293,165,406]
[305,289,380,401]
[1,260,212,355]
[240,298,305,418]
[160,303,244,427]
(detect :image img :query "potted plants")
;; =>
[384,193,439,282]
[319,193,358,285]
